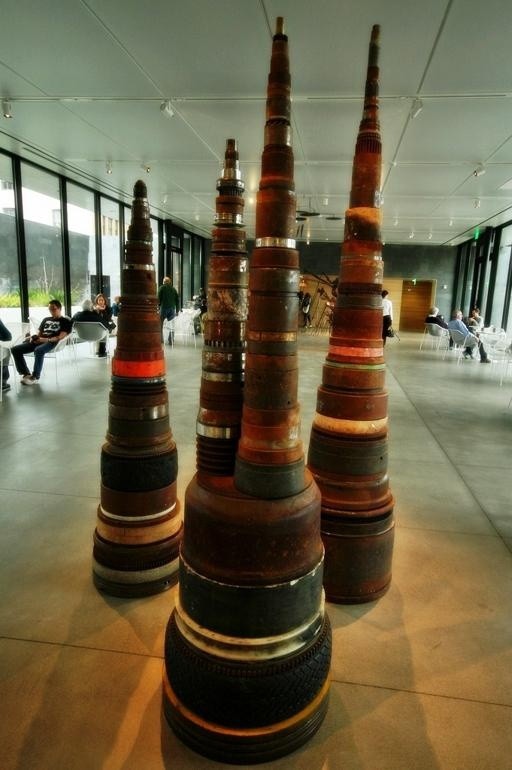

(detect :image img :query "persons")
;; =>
[0,317,12,391]
[70,300,115,357]
[301,293,313,329]
[464,308,483,359]
[112,297,122,317]
[11,299,70,385]
[93,294,112,355]
[194,288,207,335]
[299,290,303,304]
[424,307,453,349]
[447,310,492,363]
[382,289,394,348]
[157,277,181,345]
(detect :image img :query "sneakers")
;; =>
[481,359,489,363]
[21,374,40,386]
[2,383,10,391]
[462,351,474,359]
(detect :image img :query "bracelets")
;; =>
[48,337,51,342]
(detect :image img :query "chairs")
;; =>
[160,307,201,350]
[0,316,112,403]
[419,322,512,388]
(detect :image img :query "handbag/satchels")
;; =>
[387,328,394,337]
